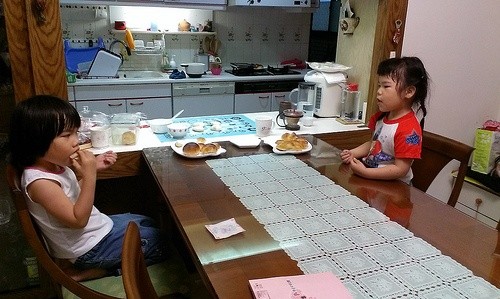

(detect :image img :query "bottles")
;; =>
[341,82,360,121]
[178,18,191,32]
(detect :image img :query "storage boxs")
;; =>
[471,129,500,175]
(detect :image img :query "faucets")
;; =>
[108,39,131,56]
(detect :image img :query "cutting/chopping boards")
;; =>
[88,51,122,78]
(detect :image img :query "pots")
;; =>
[180,63,205,77]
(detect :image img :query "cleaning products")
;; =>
[169,54,176,66]
[161,33,166,48]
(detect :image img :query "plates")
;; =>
[171,140,227,158]
[263,135,313,154]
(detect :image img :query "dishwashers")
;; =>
[171,81,236,118]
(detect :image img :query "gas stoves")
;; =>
[224,63,300,76]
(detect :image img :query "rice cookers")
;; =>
[303,70,347,117]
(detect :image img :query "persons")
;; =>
[339,55,429,186]
[7,94,172,271]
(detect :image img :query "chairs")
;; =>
[14,190,190,299]
[411,130,476,207]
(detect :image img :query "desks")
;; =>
[143,135,500,299]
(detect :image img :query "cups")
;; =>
[289,80,316,119]
[302,105,314,126]
[134,40,144,47]
[95,7,107,19]
[279,101,291,119]
[282,108,303,129]
[255,117,275,136]
[90,128,109,147]
[151,21,158,31]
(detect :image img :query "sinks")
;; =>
[123,70,164,78]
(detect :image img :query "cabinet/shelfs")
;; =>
[235,92,297,114]
[73,83,173,120]
[453,177,500,230]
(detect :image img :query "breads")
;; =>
[122,131,137,145]
[275,132,308,151]
[182,142,219,155]
[126,30,135,49]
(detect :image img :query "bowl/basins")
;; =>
[167,122,190,138]
[111,112,141,144]
[147,119,174,133]
[115,21,126,31]
